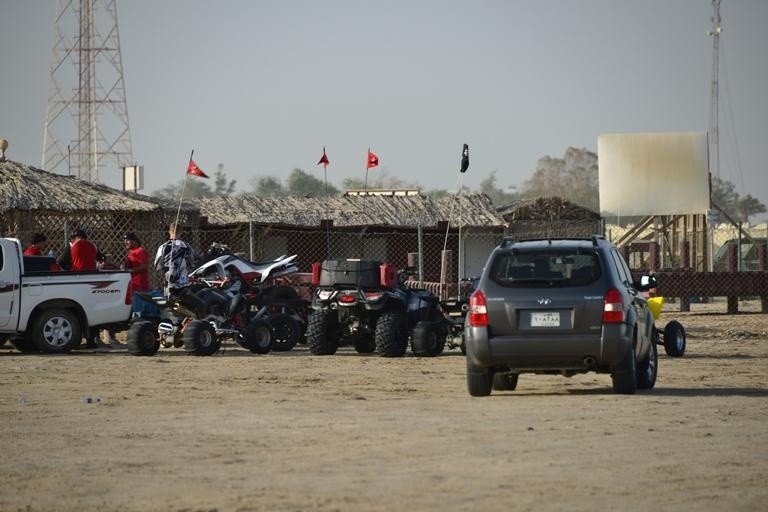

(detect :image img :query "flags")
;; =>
[460,143,470,173]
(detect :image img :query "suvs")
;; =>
[457,234,658,397]
[127,274,273,357]
[180,249,308,351]
[308,258,450,358]
[411,276,481,357]
[645,295,687,357]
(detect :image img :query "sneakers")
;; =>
[96,339,105,345]
[108,338,120,344]
[86,341,98,348]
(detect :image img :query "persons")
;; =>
[24,233,48,256]
[120,232,150,304]
[57,230,99,349]
[153,221,194,298]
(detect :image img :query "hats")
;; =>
[123,233,136,240]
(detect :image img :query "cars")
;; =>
[712,237,767,272]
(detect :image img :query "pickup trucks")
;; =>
[0,238,133,353]
